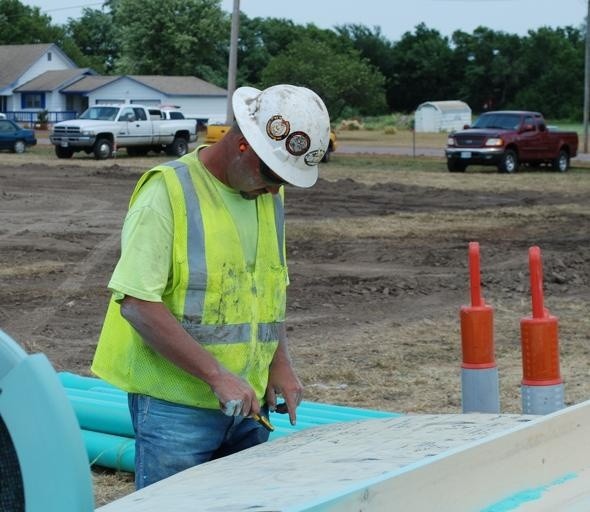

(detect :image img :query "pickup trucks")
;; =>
[48,103,199,159]
[205,124,338,164]
[443,110,581,174]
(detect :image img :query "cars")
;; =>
[0,117,37,153]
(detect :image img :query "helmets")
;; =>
[233,83,330,188]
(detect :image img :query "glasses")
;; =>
[260,159,289,185]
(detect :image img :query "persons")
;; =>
[91,83,335,495]
